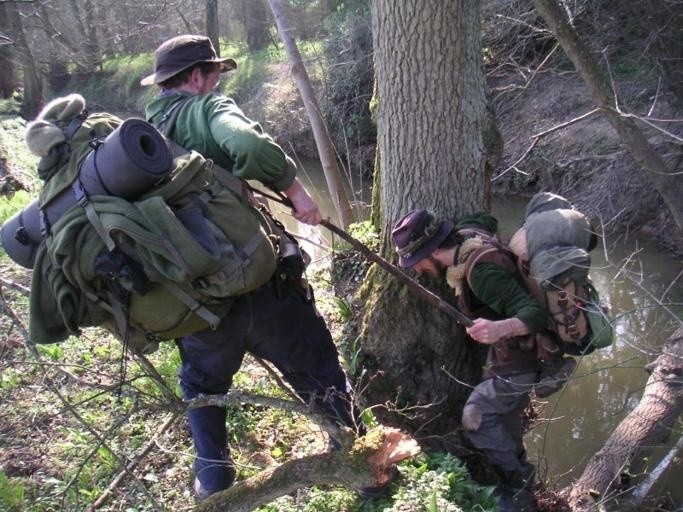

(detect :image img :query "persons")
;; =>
[140,33,402,501]
[390,208,567,511]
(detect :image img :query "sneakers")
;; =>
[494,480,540,511]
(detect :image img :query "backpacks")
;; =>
[506,187,620,360]
[1,91,283,361]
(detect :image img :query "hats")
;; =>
[140,33,238,88]
[391,208,454,269]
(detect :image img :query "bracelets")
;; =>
[505,318,514,340]
